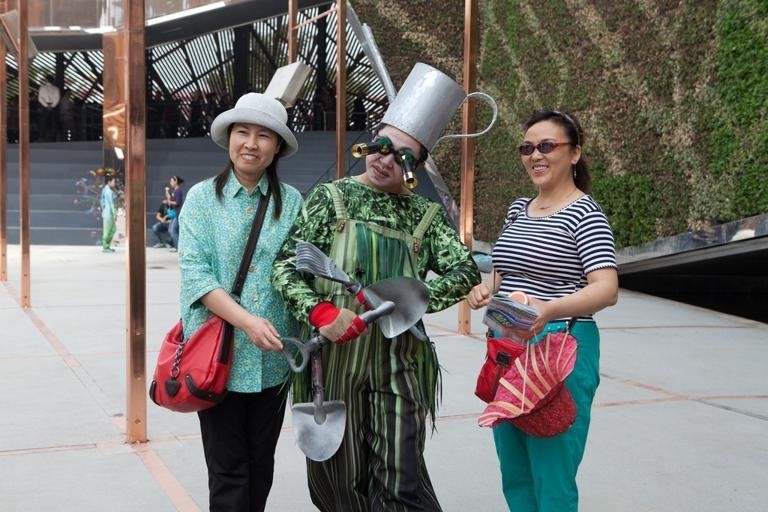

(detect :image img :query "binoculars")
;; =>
[352,136,418,189]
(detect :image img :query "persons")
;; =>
[465,111,620,512]
[150,193,175,250]
[4,72,372,141]
[111,194,128,250]
[177,90,304,512]
[266,122,483,510]
[161,175,184,253]
[99,175,119,253]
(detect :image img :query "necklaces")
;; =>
[531,186,578,210]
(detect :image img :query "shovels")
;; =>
[291,327,347,462]
[278,276,430,373]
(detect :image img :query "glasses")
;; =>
[516,140,574,155]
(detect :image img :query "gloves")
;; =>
[310,301,365,343]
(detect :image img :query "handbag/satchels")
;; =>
[149,294,235,413]
[482,294,540,342]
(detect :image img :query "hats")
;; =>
[210,92,299,162]
